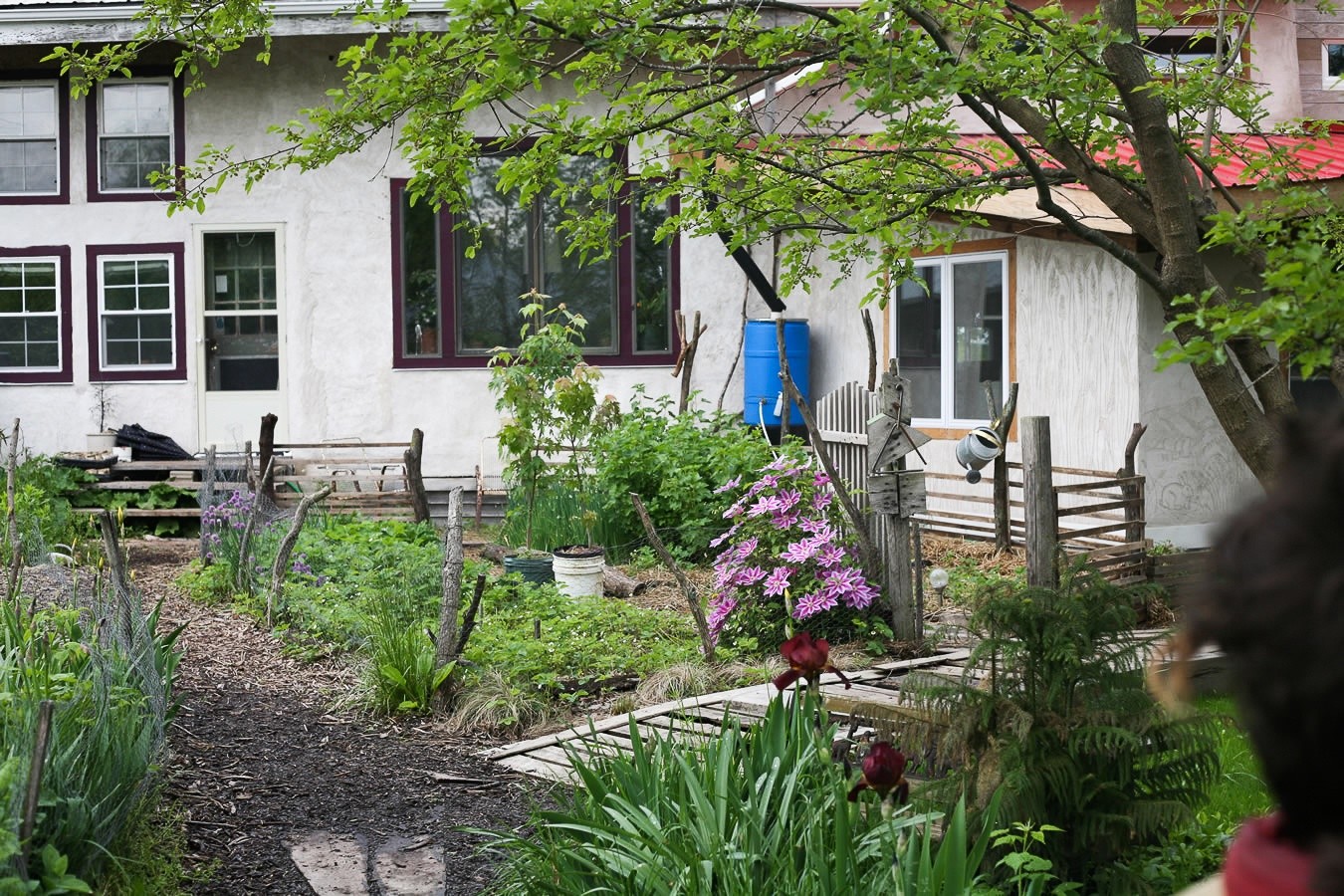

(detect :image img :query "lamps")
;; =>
[930,570,949,593]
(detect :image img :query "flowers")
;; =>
[406,268,436,328]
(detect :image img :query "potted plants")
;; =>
[486,287,624,603]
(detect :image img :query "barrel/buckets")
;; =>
[552,544,607,598]
[744,317,812,427]
[503,552,554,586]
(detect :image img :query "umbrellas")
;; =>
[108,423,197,461]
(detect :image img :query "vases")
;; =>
[420,326,435,353]
[87,433,117,452]
